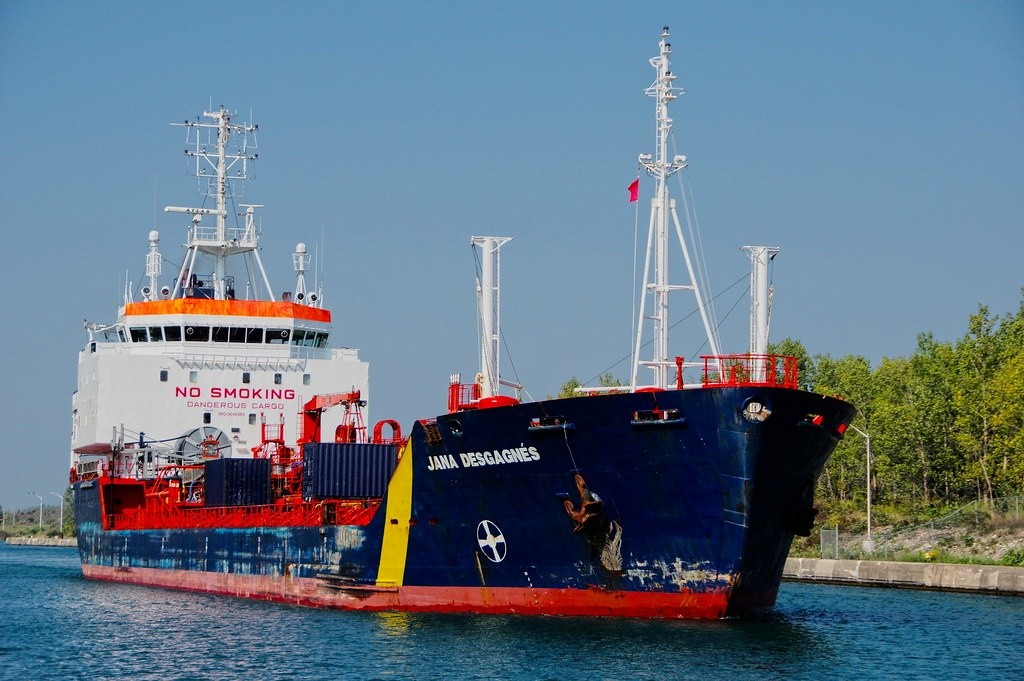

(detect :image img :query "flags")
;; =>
[628,180,639,201]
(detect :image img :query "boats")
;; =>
[69,25,858,622]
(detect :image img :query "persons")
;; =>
[565,474,626,591]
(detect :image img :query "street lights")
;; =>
[29,492,42,532]
[51,492,63,539]
